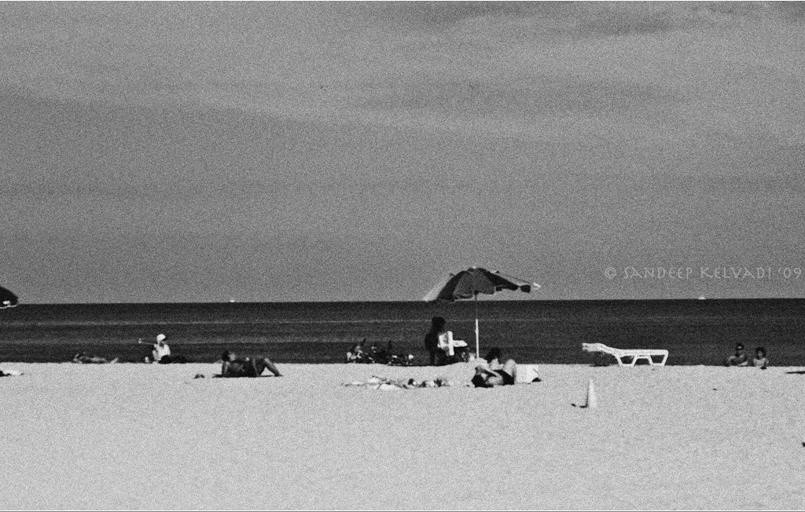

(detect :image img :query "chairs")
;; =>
[582,342,668,368]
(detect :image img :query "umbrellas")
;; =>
[421,265,541,359]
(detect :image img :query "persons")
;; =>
[726,343,749,368]
[470,358,518,387]
[149,334,170,364]
[751,347,771,368]
[220,350,284,378]
[345,344,415,368]
[426,315,469,367]
